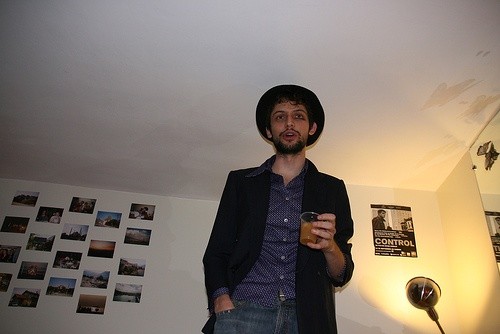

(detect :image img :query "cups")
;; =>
[299,212,321,245]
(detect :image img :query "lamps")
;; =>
[404,275,445,334]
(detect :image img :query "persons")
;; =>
[494,215,500,237]
[0,192,153,314]
[372,209,389,231]
[201,84,355,334]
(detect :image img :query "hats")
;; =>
[256,85,325,146]
